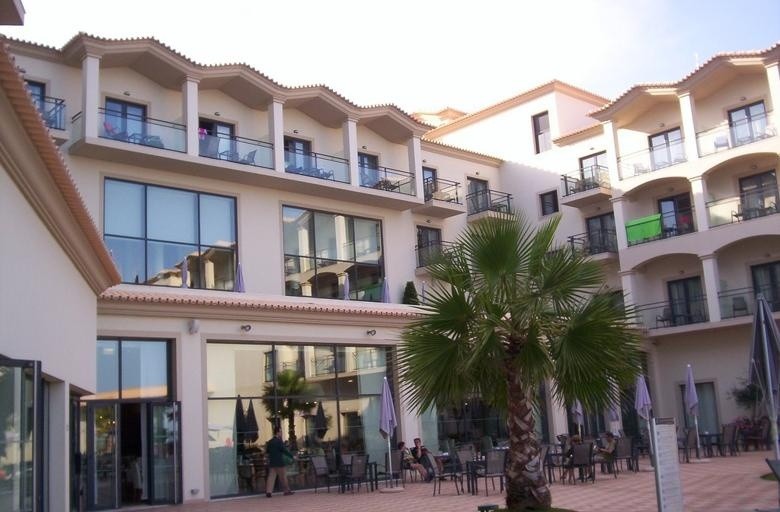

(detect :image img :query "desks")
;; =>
[219,150,239,161]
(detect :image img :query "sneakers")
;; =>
[266,493,272,497]
[283,491,295,496]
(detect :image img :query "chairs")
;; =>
[733,296,748,318]
[236,450,307,493]
[730,204,773,223]
[285,163,335,179]
[308,415,650,498]
[240,147,257,165]
[470,197,507,211]
[662,223,678,238]
[655,308,673,328]
[713,134,729,152]
[675,416,770,463]
[360,172,400,192]
[130,461,145,503]
[104,120,164,148]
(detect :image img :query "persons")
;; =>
[398,441,434,484]
[581,430,616,477]
[411,437,448,481]
[262,424,295,498]
[556,434,587,482]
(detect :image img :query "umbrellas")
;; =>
[632,365,655,457]
[569,398,585,438]
[243,398,259,449]
[604,375,620,424]
[745,291,780,462]
[684,364,703,451]
[378,378,398,440]
[231,393,245,443]
[313,403,327,439]
